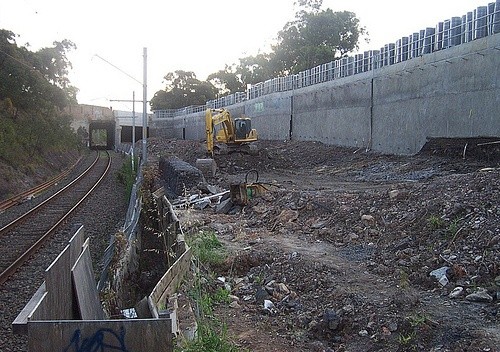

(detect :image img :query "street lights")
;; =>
[92,46,149,166]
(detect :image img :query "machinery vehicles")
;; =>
[196,109,258,179]
